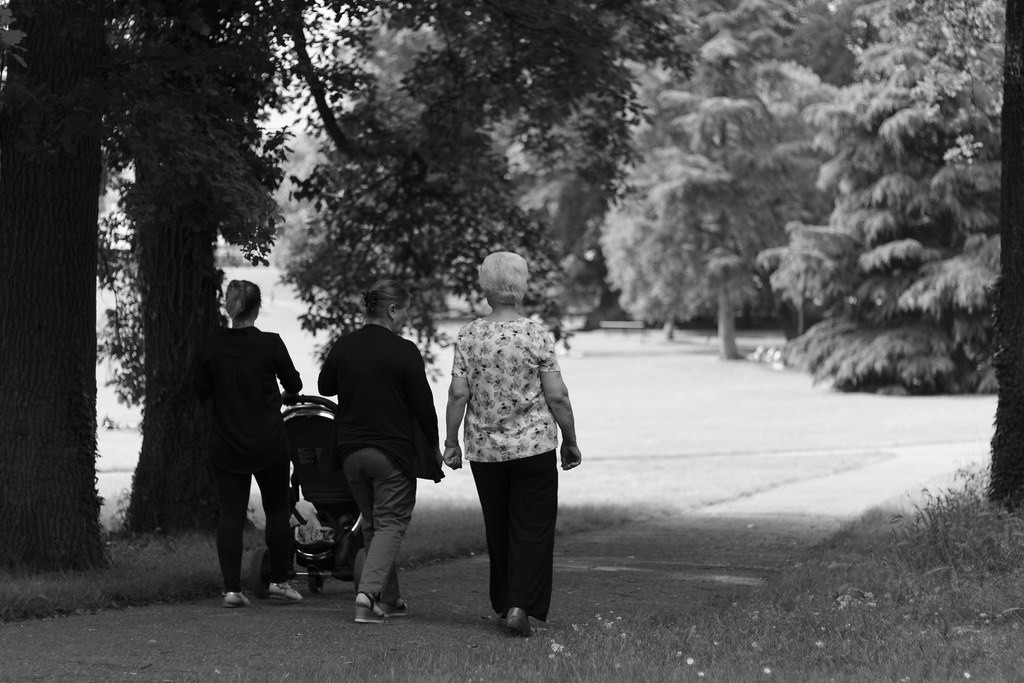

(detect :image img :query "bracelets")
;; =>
[445,441,459,448]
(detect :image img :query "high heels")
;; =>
[379,595,408,618]
[353,591,385,625]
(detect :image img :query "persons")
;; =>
[317,281,445,624]
[441,252,582,636]
[190,279,303,611]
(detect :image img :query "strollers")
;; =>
[273,395,389,606]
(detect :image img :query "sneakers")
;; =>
[267,582,303,601]
[221,593,250,608]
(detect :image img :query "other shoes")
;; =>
[507,607,530,636]
